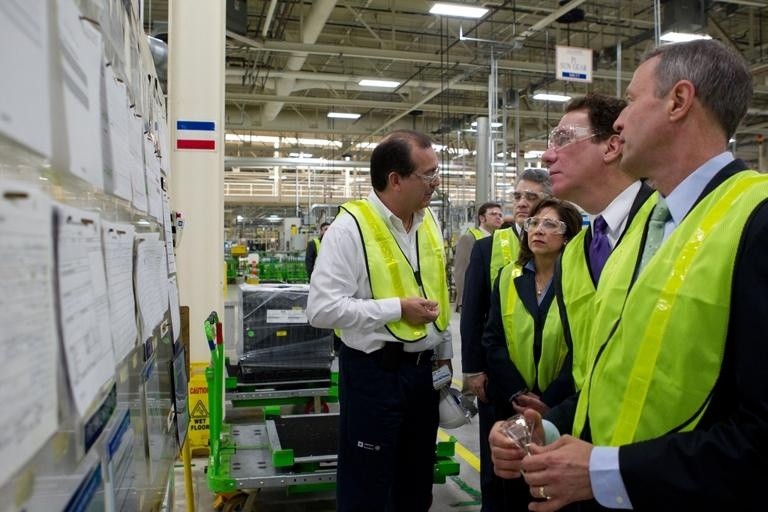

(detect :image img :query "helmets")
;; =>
[439,387,477,429]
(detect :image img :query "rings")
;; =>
[537,484,544,496]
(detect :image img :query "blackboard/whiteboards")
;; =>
[266,411,340,463]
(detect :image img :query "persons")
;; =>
[460,168,554,512]
[488,38,768,512]
[478,198,584,414]
[305,222,332,284]
[541,93,657,416]
[305,129,454,512]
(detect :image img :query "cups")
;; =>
[496,413,536,457]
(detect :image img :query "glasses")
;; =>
[416,166,440,182]
[523,216,566,235]
[545,125,606,152]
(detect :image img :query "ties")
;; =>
[589,216,611,287]
[638,202,669,278]
